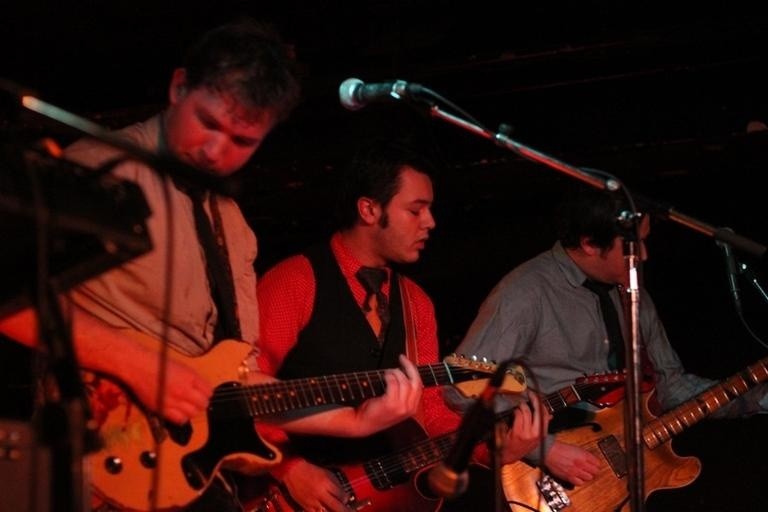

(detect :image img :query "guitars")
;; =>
[500,355,768,506]
[74,327,521,512]
[224,367,645,511]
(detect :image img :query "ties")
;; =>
[582,279,626,369]
[355,265,389,346]
[171,179,230,341]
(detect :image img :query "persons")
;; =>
[452,190,768,512]
[255,147,555,511]
[61,38,424,511]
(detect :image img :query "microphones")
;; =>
[338,78,409,111]
[426,377,510,499]
